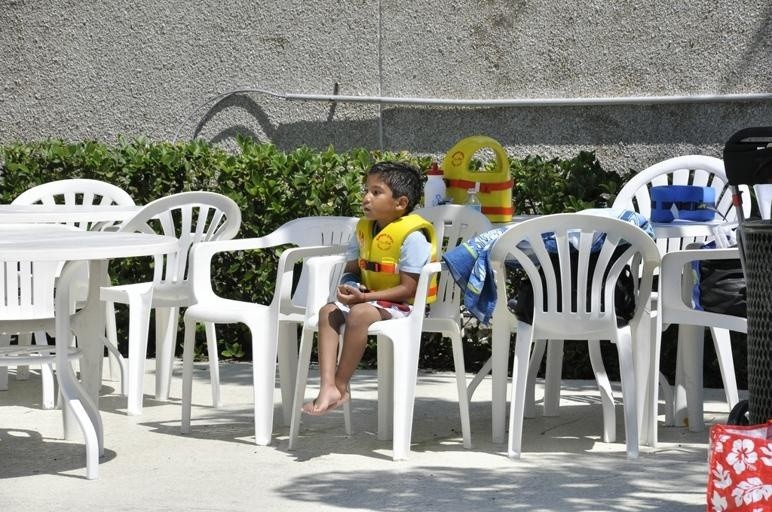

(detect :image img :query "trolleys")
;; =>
[721,125,772,426]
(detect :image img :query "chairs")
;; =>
[180,216,359,448]
[66,191,241,415]
[649,184,772,453]
[490,212,660,460]
[287,205,497,461]
[613,154,752,430]
[10,177,136,408]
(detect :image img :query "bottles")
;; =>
[423,162,447,208]
[462,180,481,214]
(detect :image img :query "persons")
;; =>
[301,161,438,417]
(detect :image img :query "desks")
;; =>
[0,203,141,402]
[1,223,181,480]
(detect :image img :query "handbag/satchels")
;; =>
[517,246,636,328]
[698,244,747,315]
[706,420,772,511]
